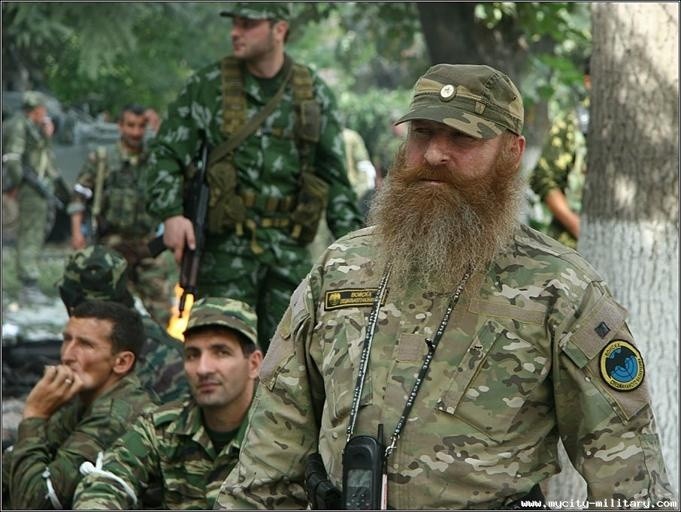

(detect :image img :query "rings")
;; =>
[65,379,72,386]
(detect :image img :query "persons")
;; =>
[1,90,193,406]
[211,62,676,511]
[143,2,367,356]
[529,56,590,253]
[71,294,265,511]
[1,300,156,510]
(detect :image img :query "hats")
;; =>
[23,90,50,108]
[182,298,259,345]
[53,245,128,303]
[220,3,291,23]
[394,62,524,141]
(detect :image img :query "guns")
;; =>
[22,163,65,210]
[146,141,210,317]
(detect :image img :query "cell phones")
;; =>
[340,422,388,510]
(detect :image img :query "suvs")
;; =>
[0,85,158,243]
